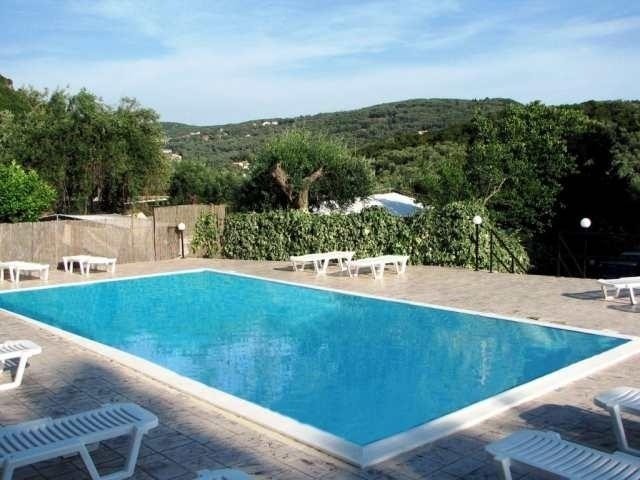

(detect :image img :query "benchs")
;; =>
[0,339,42,390]
[0,260,51,283]
[484,428,640,480]
[288,249,356,277]
[340,254,409,280]
[0,399,159,480]
[592,385,640,456]
[62,254,117,276]
[596,276,640,304]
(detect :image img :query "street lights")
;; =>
[580,218,592,278]
[473,216,482,271]
[178,222,185,258]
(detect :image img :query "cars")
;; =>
[599,251,640,275]
